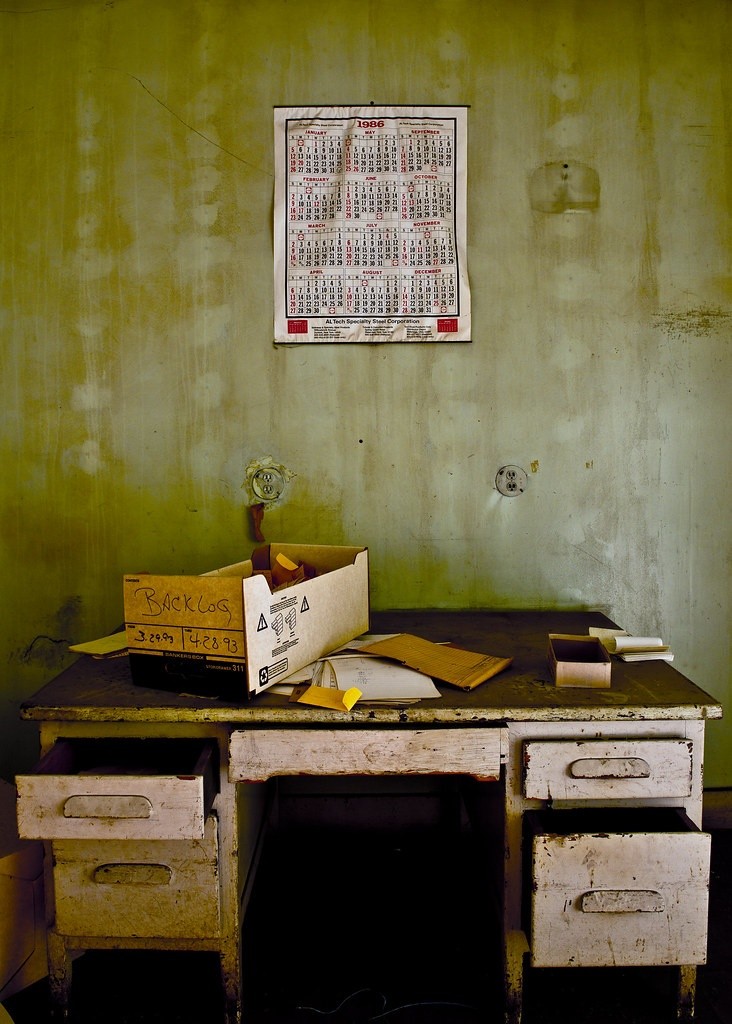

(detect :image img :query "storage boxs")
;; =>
[545,632,612,688]
[122,542,370,706]
[0,776,86,1001]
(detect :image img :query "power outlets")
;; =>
[495,464,528,497]
[251,468,285,500]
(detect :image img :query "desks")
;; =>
[16,612,721,1024]
